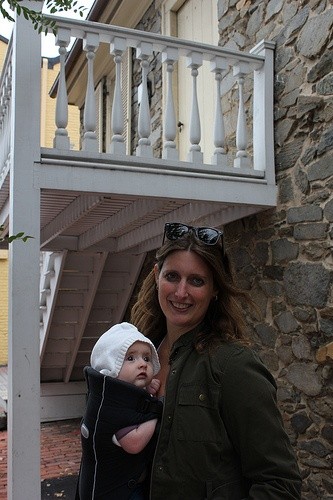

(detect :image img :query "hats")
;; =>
[89,321,161,377]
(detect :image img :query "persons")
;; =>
[130,222,304,500]
[74,323,163,500]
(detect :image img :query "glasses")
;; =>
[162,222,225,256]
